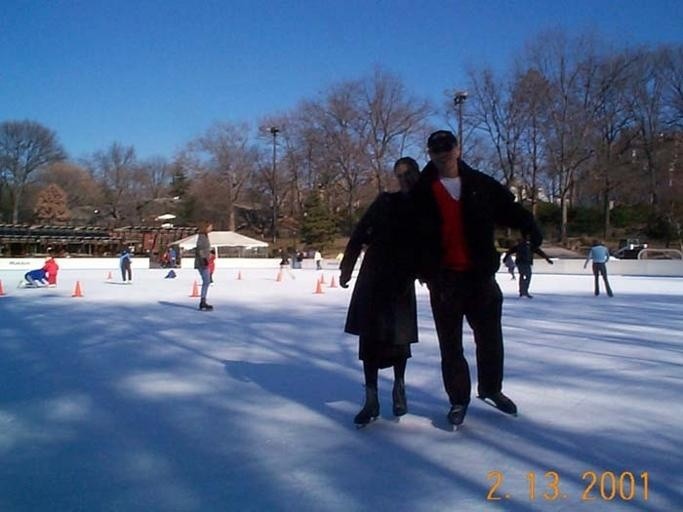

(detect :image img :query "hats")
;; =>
[426,129,458,153]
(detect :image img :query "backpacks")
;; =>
[123,258,130,270]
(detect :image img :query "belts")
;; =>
[594,262,604,265]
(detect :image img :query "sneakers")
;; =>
[595,291,599,295]
[447,399,470,425]
[519,291,533,298]
[477,381,516,415]
[607,292,613,297]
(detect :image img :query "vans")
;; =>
[146,243,184,268]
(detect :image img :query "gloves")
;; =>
[338,270,352,289]
[503,255,509,262]
[546,259,553,265]
[416,261,439,282]
[519,220,543,249]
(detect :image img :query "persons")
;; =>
[43,254,59,289]
[277,246,295,280]
[168,246,175,268]
[119,248,133,284]
[501,232,552,298]
[404,130,541,431]
[158,249,168,268]
[16,268,48,287]
[208,250,215,284]
[338,157,438,430]
[193,220,213,310]
[583,238,613,297]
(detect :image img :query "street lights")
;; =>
[270,126,280,243]
[454,91,469,160]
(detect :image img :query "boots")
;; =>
[391,380,408,416]
[199,299,213,309]
[353,386,379,424]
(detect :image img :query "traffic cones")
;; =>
[189,280,201,297]
[312,274,338,294]
[0,278,7,296]
[276,272,283,281]
[72,280,84,298]
[235,271,243,280]
[107,271,113,279]
